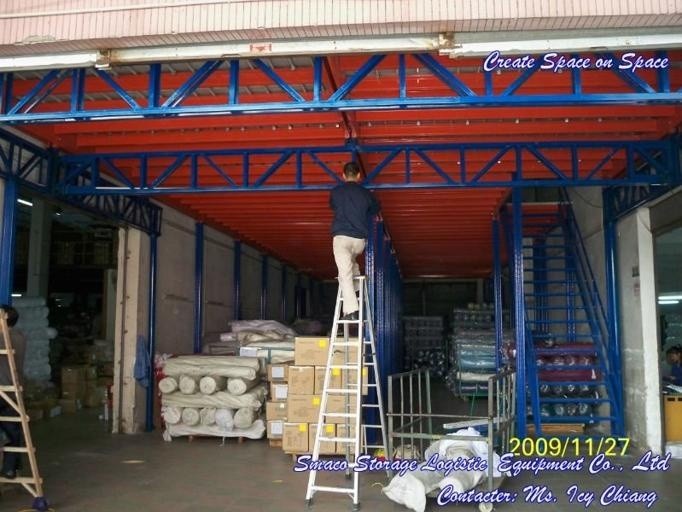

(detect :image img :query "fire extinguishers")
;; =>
[106,383,112,408]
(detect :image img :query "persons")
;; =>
[661,348,676,378]
[329,161,382,320]
[1,302,29,479]
[662,343,681,387]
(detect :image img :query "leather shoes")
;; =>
[1,468,17,479]
[339,313,358,321]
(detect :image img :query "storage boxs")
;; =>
[265,333,369,460]
[23,387,102,422]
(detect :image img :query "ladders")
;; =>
[0,308,47,512]
[304,274,393,511]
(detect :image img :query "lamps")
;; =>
[656,292,681,305]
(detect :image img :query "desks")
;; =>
[663,393,682,443]
